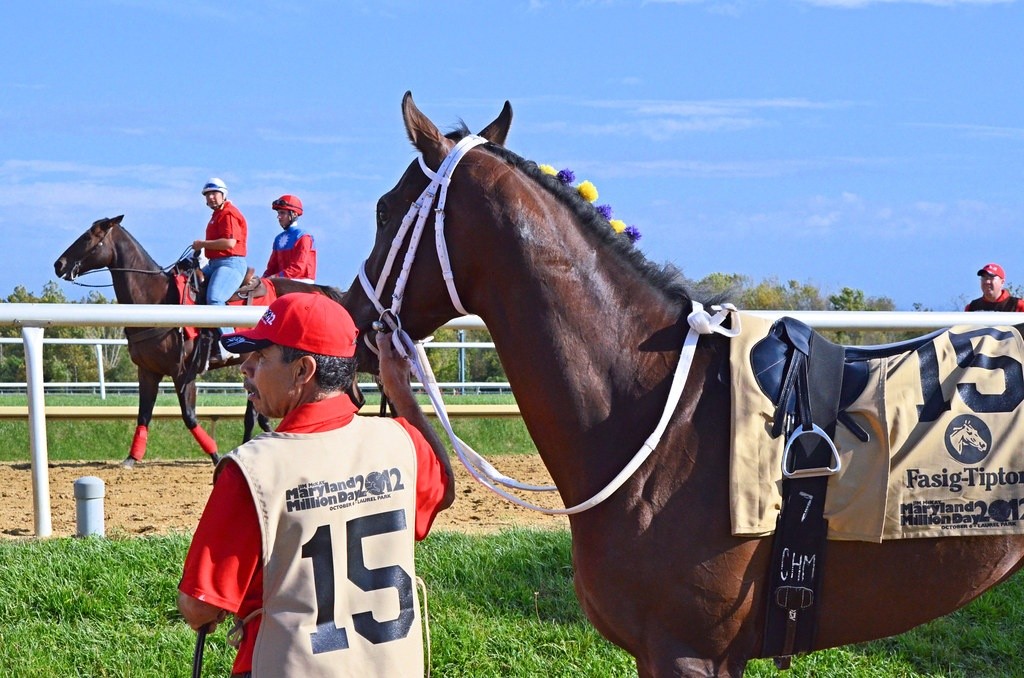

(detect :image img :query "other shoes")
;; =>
[210,353,222,363]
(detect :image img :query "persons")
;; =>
[260,194,319,283]
[176,290,457,678]
[964,262,1024,312]
[190,175,247,363]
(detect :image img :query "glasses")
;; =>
[204,183,218,189]
[273,200,287,207]
[981,275,998,281]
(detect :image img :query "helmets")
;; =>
[272,195,303,215]
[202,178,227,200]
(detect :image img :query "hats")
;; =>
[220,291,359,358]
[977,264,1005,279]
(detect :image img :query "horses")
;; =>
[343,90,1024,678]
[55,215,364,473]
